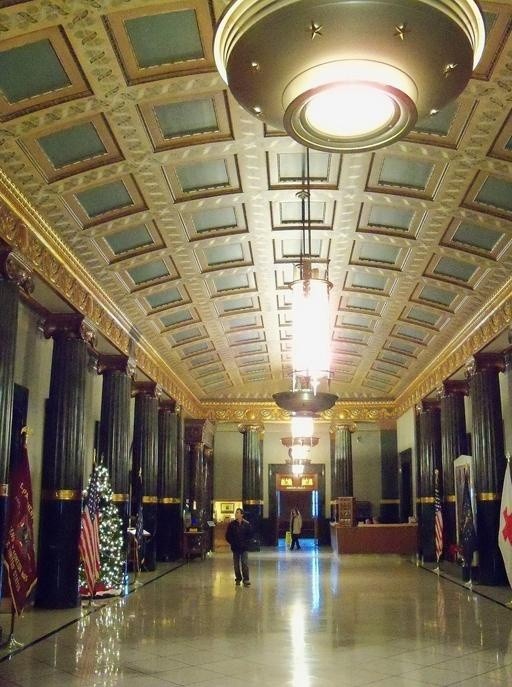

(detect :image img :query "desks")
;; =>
[185,532,208,561]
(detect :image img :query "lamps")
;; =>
[211,0,487,155]
[273,191,337,416]
[272,418,339,477]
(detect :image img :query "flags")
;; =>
[498,462,512,587]
[433,479,443,558]
[78,471,101,597]
[133,476,145,569]
[460,482,476,566]
[0,446,39,618]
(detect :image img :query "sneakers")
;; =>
[236,580,251,586]
[290,547,301,550]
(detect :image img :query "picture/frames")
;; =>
[221,503,234,513]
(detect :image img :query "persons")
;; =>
[364,513,382,523]
[226,507,253,585]
[288,506,303,550]
